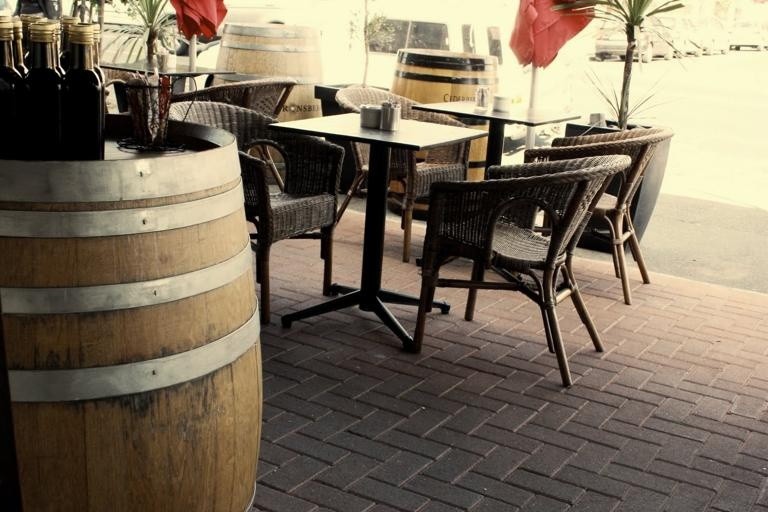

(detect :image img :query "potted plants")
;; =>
[100,0,187,112]
[547,0,674,255]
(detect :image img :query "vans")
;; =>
[366,8,503,90]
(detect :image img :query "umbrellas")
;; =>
[168,0,227,91]
[505,0,596,163]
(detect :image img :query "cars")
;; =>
[152,0,285,56]
[595,25,768,63]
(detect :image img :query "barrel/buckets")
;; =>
[213,23,324,163]
[389,48,498,220]
[0,112,263,512]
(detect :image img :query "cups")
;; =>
[162,55,177,70]
[380,107,401,132]
[493,96,508,113]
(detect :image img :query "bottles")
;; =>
[360,105,381,128]
[0,12,106,160]
[476,88,489,107]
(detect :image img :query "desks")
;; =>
[98,61,238,113]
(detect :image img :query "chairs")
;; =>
[523,126,673,305]
[335,86,473,264]
[167,101,345,326]
[411,154,632,386]
[171,77,297,191]
